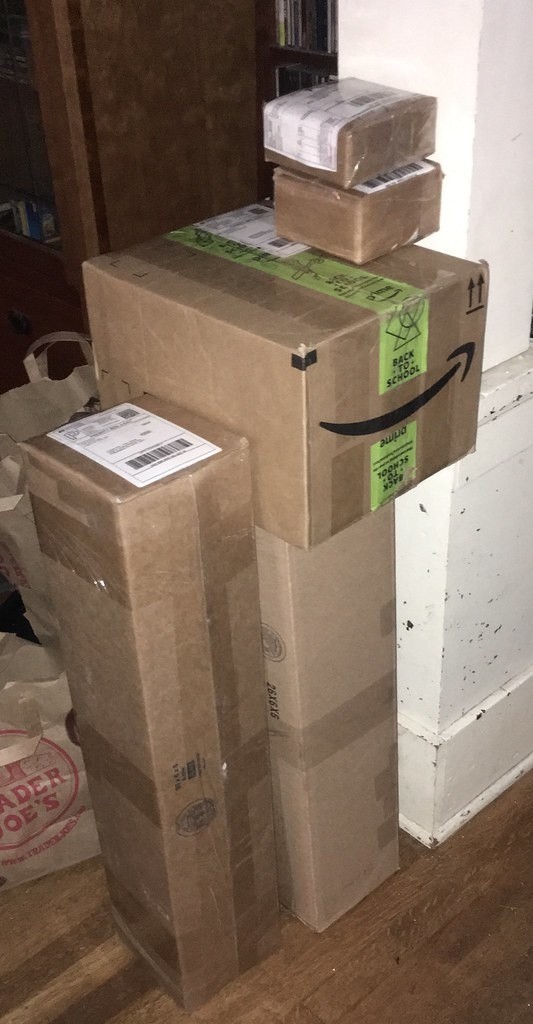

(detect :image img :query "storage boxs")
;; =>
[14,394,282,1011]
[255,501,401,934]
[260,76,438,190]
[80,199,490,552]
[273,158,443,265]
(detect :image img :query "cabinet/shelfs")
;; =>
[0,0,336,387]
